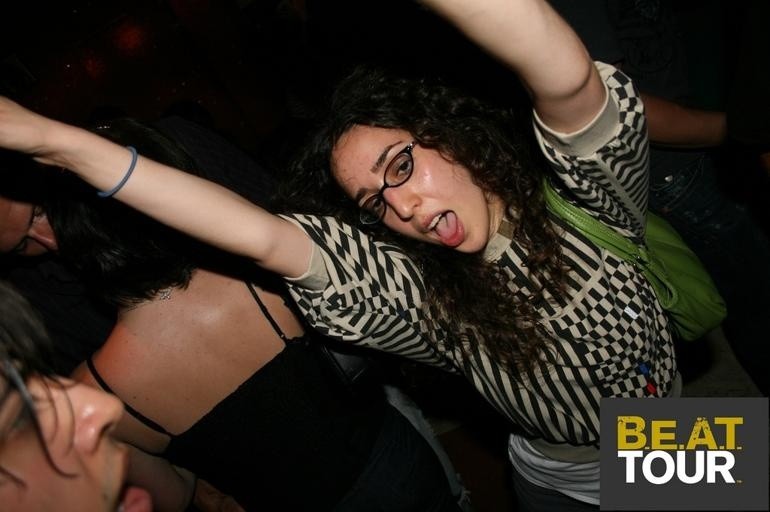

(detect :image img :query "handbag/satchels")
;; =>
[543,175,729,342]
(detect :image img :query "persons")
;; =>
[0,0,770,512]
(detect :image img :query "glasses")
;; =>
[359,140,416,225]
